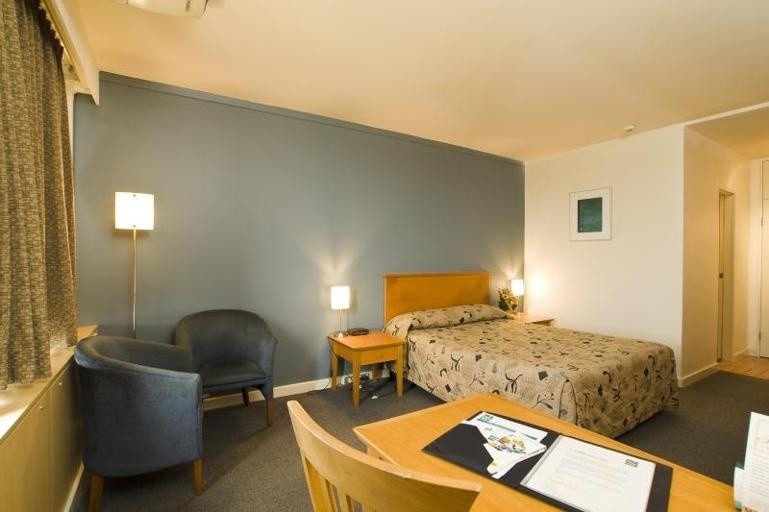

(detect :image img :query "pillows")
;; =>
[384,304,514,340]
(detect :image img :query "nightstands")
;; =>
[513,314,553,328]
[327,329,407,408]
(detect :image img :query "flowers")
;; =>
[498,288,518,313]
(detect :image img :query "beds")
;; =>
[384,274,679,437]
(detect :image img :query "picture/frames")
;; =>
[570,186,613,239]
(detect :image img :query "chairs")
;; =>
[286,400,480,512]
[73,337,203,511]
[175,309,277,426]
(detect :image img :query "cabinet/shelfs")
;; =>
[0,357,81,512]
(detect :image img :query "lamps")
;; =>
[511,280,525,316]
[330,285,351,337]
[114,191,156,337]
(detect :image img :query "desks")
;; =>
[352,390,769,512]
